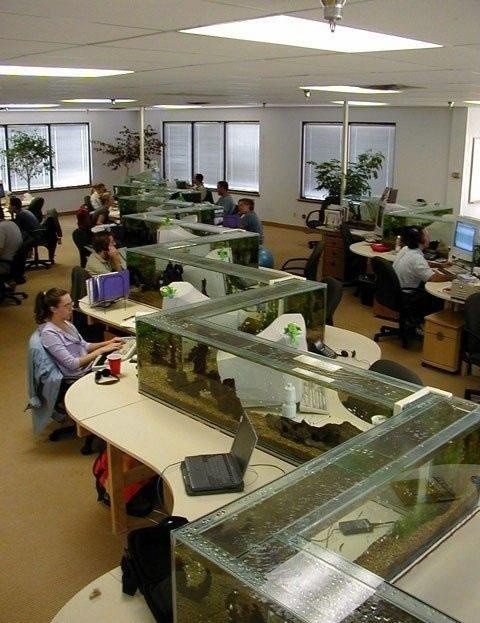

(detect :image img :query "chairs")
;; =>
[70,194,104,341]
[281,241,323,280]
[369,256,426,349]
[306,196,338,248]
[25,321,84,441]
[367,360,423,387]
[0,196,58,305]
[460,294,480,376]
[205,187,212,201]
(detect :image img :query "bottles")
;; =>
[395,236,401,251]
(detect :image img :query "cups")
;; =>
[107,353,121,376]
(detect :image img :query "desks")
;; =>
[349,226,480,313]
[389,509,480,623]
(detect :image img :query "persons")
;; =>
[238,198,263,245]
[85,231,122,277]
[214,180,236,214]
[90,184,114,233]
[193,174,207,201]
[7,197,40,239]
[392,225,456,333]
[0,206,23,276]
[33,287,126,393]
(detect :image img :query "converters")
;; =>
[339,519,374,535]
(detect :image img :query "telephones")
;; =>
[310,339,338,359]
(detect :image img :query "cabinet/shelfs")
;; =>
[422,313,464,374]
[319,229,369,284]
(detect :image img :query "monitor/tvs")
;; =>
[0,183,5,199]
[374,202,407,236]
[449,216,480,273]
[159,280,211,311]
[214,313,308,408]
[177,247,233,299]
[154,224,211,272]
[179,214,198,234]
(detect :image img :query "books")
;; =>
[323,203,350,229]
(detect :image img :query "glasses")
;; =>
[56,301,74,309]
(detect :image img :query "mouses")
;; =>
[131,354,137,362]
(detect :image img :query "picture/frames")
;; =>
[468,137,480,203]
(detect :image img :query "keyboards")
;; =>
[104,338,136,365]
[362,233,382,243]
[78,295,89,305]
[297,381,332,415]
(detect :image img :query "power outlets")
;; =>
[301,214,306,218]
[291,211,298,218]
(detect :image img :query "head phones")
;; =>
[335,349,356,358]
[409,228,424,244]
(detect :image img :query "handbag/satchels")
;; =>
[120,516,189,623]
[92,444,163,518]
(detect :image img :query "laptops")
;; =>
[181,410,258,495]
